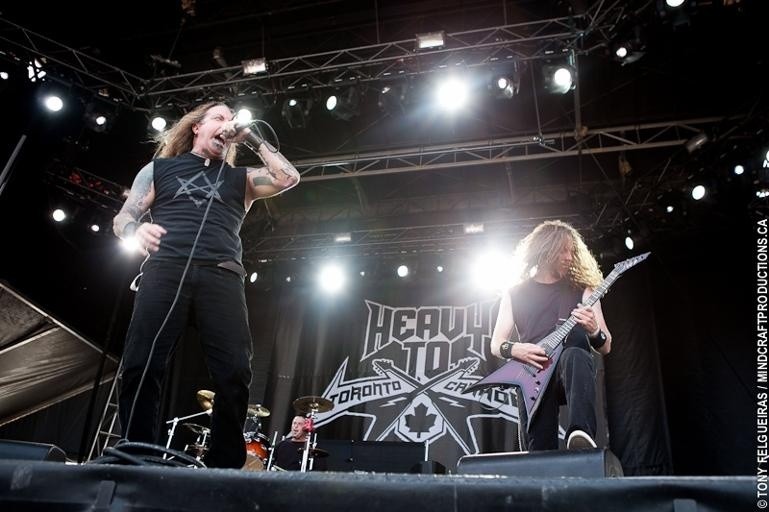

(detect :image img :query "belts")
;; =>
[218,261,246,275]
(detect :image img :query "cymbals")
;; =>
[196,390,215,410]
[247,404,271,418]
[298,447,330,458]
[293,396,333,413]
[178,423,210,436]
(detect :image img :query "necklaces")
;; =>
[190,151,217,166]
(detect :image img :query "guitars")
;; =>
[461,251,652,434]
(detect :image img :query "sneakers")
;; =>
[567,427,597,450]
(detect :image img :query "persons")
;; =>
[271,414,313,471]
[490,221,613,453]
[112,101,301,474]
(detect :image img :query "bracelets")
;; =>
[241,130,265,154]
[500,340,514,359]
[588,328,607,350]
[121,221,142,240]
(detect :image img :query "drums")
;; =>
[242,432,272,471]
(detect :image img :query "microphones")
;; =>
[221,119,254,138]
